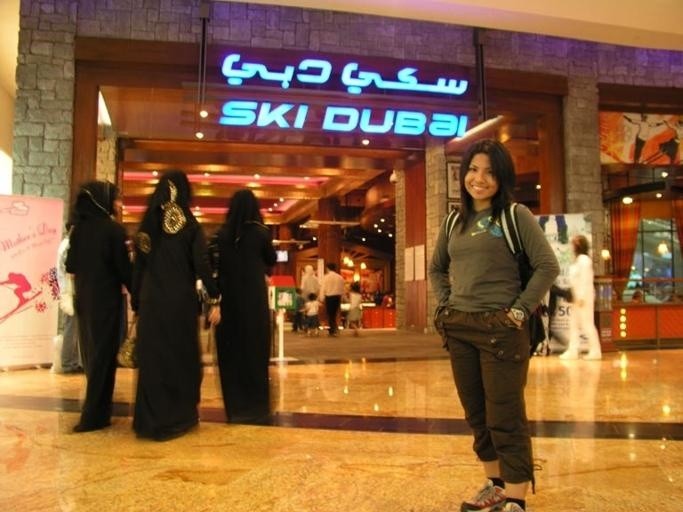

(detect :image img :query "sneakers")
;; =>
[459,478,508,512]
[498,500,526,512]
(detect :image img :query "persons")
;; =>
[290,263,394,338]
[66,179,140,432]
[208,190,277,424]
[58,219,84,373]
[629,292,643,302]
[131,169,223,442]
[559,234,602,360]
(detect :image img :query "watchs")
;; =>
[428,139,561,512]
[510,308,525,320]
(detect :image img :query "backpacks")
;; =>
[445,198,551,360]
